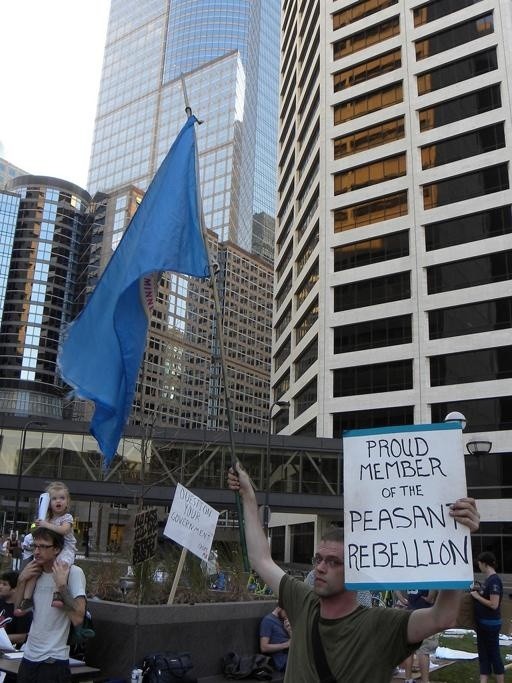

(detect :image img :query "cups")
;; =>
[310,556,345,568]
[29,544,54,550]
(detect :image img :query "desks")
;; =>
[259,400,293,542]
[444,411,468,433]
[7,420,49,554]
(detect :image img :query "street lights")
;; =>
[142,650,196,683]
[221,650,273,683]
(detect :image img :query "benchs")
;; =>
[57,114,213,470]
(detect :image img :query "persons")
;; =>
[371,589,438,683]
[0,524,37,569]
[1,572,32,647]
[14,526,87,683]
[260,604,291,671]
[305,569,371,608]
[228,462,481,683]
[15,482,76,615]
[204,552,224,590]
[471,553,504,683]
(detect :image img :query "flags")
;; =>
[57,114,213,470]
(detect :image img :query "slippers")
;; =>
[70,608,94,657]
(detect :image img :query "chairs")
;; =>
[14,598,34,617]
[51,591,64,608]
